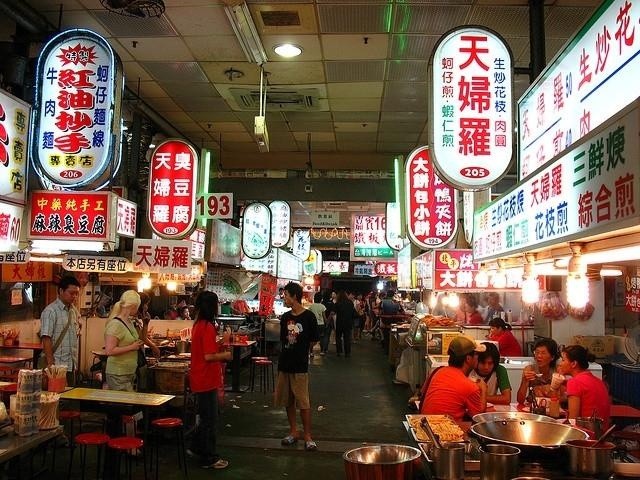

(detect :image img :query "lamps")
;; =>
[494,259,507,288]
[476,263,489,288]
[521,253,540,304]
[273,43,304,58]
[566,242,590,308]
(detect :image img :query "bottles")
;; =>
[219,322,224,333]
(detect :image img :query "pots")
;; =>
[473,411,560,423]
[470,420,590,457]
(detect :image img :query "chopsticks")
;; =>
[44,365,67,378]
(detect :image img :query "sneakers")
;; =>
[202,458,228,469]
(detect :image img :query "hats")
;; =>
[447,335,486,357]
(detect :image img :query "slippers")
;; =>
[305,440,317,451]
[281,435,298,445]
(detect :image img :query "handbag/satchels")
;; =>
[36,350,49,370]
[138,348,148,368]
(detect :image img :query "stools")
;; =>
[151,418,187,479]
[250,356,269,392]
[102,415,137,468]
[58,410,82,447]
[107,436,147,480]
[68,432,110,480]
[0,366,20,393]
[251,360,275,395]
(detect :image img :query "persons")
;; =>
[184,291,233,469]
[306,282,512,360]
[557,346,610,440]
[486,318,522,357]
[38,276,79,387]
[276,283,321,451]
[416,335,488,438]
[516,339,567,407]
[136,292,159,391]
[176,305,191,321]
[104,291,140,393]
[467,342,512,405]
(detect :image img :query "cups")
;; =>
[48,377,67,393]
[566,439,615,475]
[550,373,565,391]
[477,444,520,480]
[40,400,59,429]
[10,395,16,418]
[434,445,465,480]
